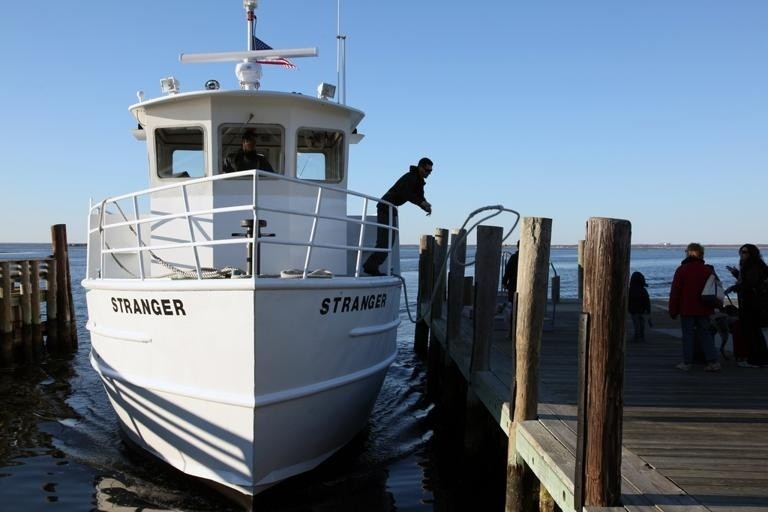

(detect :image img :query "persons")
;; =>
[628,271,652,343]
[669,243,723,374]
[723,243,768,369]
[502,240,520,341]
[220,130,274,173]
[362,157,433,276]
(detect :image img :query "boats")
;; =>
[79,0,406,512]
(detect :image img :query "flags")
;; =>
[251,35,297,69]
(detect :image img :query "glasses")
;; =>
[423,167,432,173]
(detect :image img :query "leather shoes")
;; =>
[364,264,387,276]
[362,257,371,268]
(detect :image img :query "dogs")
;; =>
[708,304,741,362]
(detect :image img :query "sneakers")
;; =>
[675,362,695,368]
[702,362,720,372]
[736,360,760,368]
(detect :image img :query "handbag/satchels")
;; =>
[699,265,724,308]
